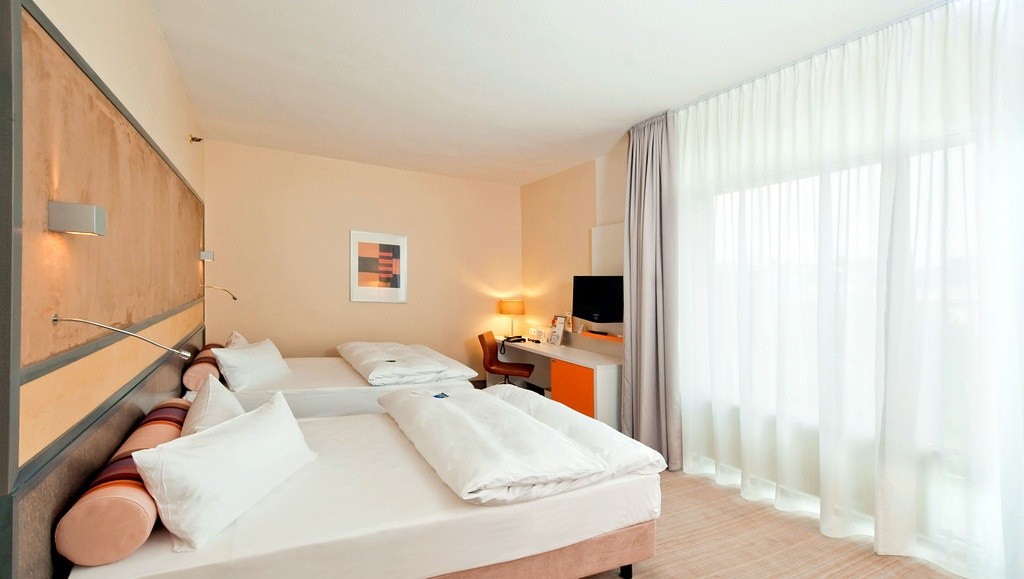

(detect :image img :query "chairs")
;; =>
[478,331,535,386]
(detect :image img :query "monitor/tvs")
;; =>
[572,275,624,324]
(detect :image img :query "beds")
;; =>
[0,323,669,579]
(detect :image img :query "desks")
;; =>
[485,335,625,432]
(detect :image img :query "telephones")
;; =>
[505,336,525,342]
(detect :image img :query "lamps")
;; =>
[52,312,191,360]
[498,300,525,338]
[200,251,215,261]
[200,284,237,300]
[46,202,107,238]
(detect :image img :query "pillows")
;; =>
[131,390,319,552]
[182,343,226,392]
[225,330,249,347]
[209,339,290,391]
[181,374,245,439]
[53,398,190,566]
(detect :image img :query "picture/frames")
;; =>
[350,231,408,303]
